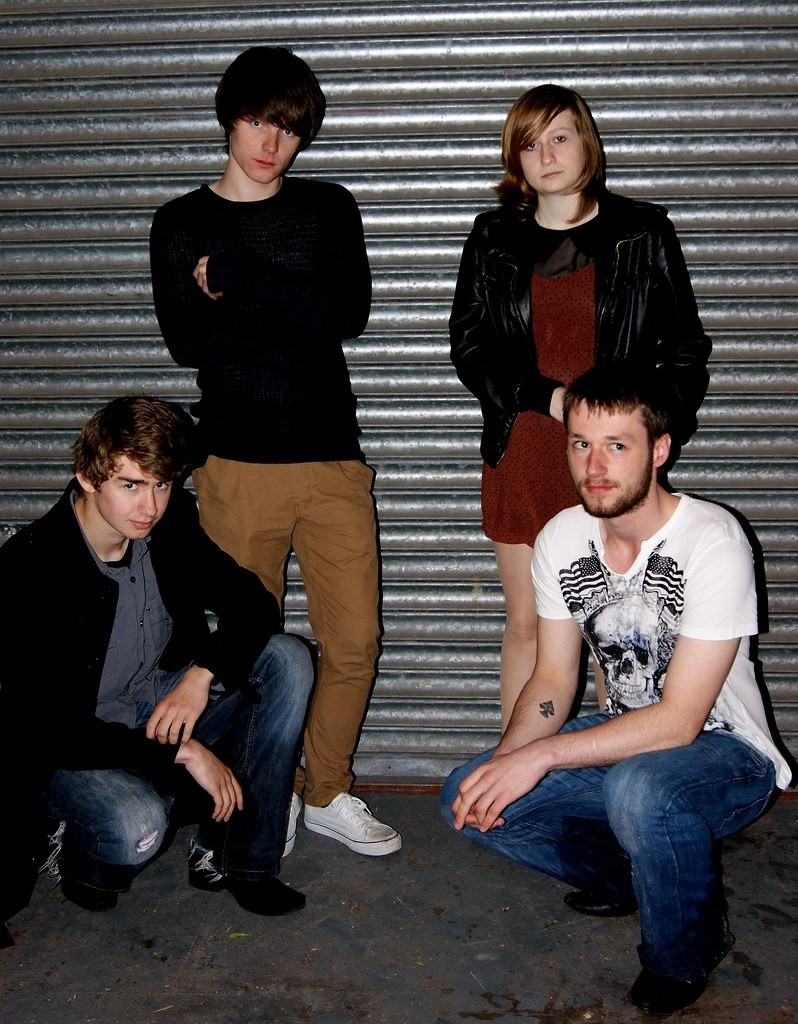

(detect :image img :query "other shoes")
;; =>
[187,834,305,916]
[59,868,119,912]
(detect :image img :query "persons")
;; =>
[148,49,413,864]
[449,82,715,750]
[4,395,317,917]
[438,359,794,1016]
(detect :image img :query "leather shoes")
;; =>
[563,863,640,918]
[628,929,728,1015]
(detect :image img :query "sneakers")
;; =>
[305,791,401,856]
[282,791,304,857]
